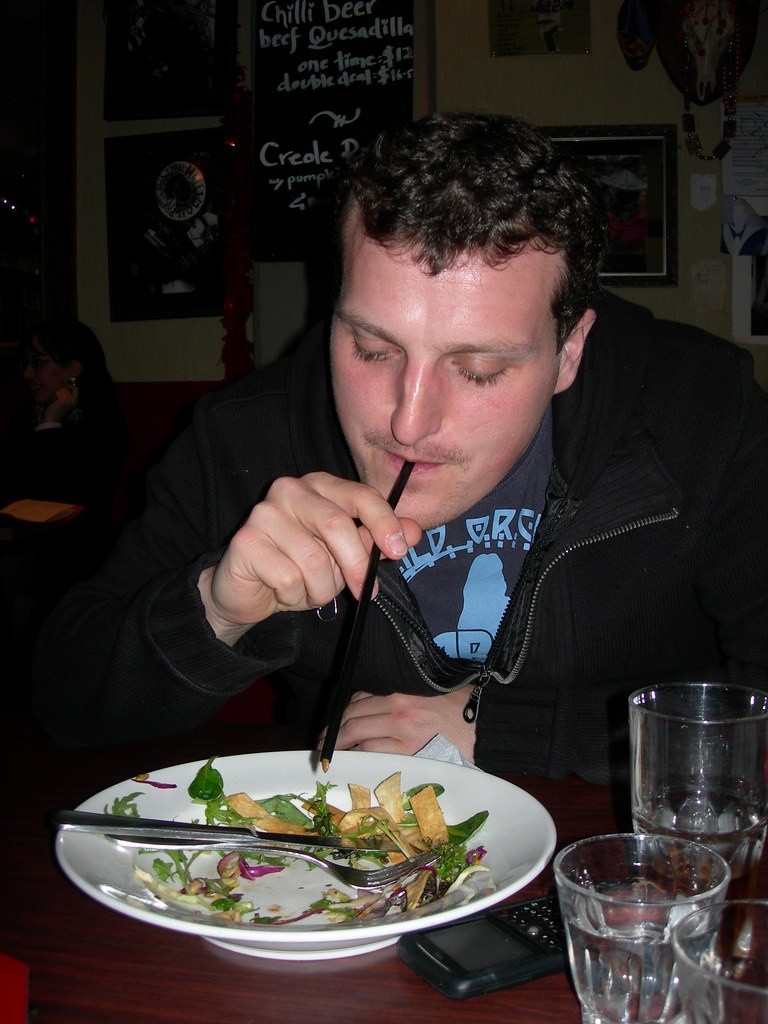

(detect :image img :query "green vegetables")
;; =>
[103,754,494,923]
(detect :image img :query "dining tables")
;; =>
[0,719,768,1024]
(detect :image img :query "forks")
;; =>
[105,833,439,891]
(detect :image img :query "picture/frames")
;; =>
[538,124,678,288]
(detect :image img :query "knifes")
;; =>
[50,808,419,853]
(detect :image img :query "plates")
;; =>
[54,751,558,962]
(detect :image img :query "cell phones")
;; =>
[398,893,570,998]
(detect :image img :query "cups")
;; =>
[554,832,731,1023]
[627,681,768,879]
[670,899,767,1024]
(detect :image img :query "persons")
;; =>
[34,112,768,788]
[0,317,128,584]
